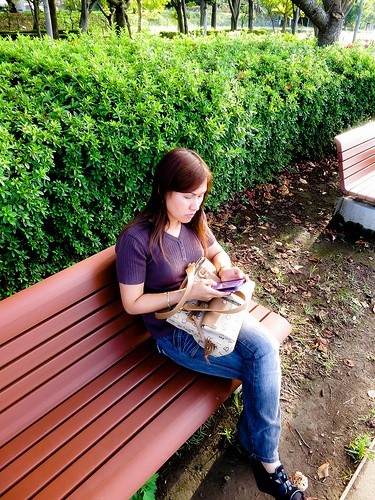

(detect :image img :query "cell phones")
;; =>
[211,278,246,292]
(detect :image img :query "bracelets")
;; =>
[217,265,227,279]
[167,291,172,310]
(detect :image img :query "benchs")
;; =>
[0,246,293,500]
[333,120,375,230]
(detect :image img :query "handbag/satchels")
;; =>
[154,257,256,357]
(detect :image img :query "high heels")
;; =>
[254,460,307,500]
[235,435,259,466]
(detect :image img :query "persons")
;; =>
[115,146,306,500]
